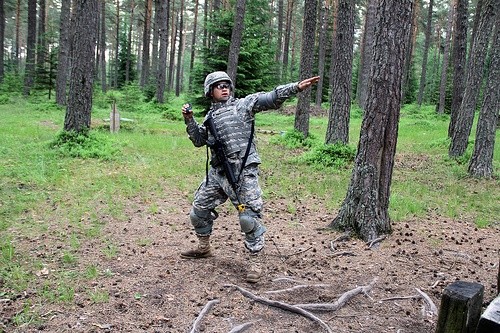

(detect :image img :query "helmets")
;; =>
[204,71,232,97]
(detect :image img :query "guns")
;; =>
[203,118,244,206]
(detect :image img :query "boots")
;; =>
[246,248,267,282]
[180,236,212,258]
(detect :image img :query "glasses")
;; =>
[212,83,231,90]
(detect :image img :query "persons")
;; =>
[180,71,320,283]
